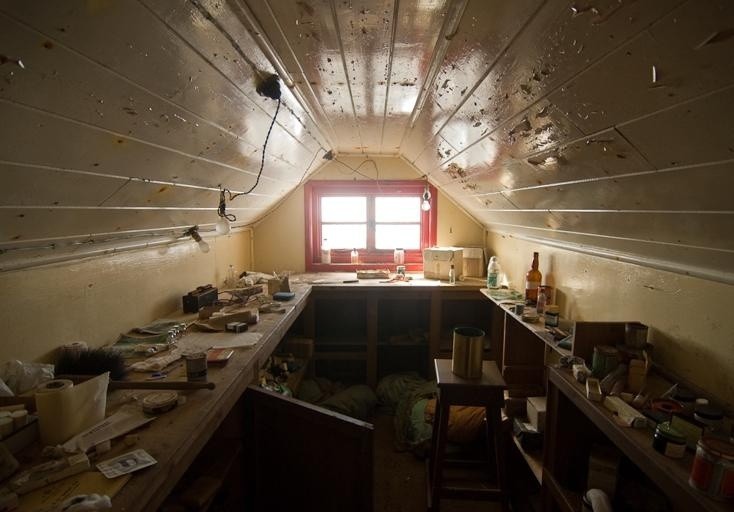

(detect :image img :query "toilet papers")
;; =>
[35,371,112,444]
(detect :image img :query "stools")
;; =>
[422,359,510,512]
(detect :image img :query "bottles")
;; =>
[524,250,543,306]
[448,265,456,284]
[536,289,546,313]
[350,246,359,264]
[485,255,502,289]
[394,247,405,265]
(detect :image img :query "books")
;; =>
[357,268,391,279]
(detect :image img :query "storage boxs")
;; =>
[525,396,547,430]
[422,246,464,283]
[460,244,494,277]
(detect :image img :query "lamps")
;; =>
[182,223,209,252]
[216,189,237,236]
[418,174,432,213]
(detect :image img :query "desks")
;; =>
[540,361,733,512]
[289,273,498,389]
[477,281,641,511]
[1,283,375,511]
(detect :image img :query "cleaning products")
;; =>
[351,248,359,264]
[449,264,455,283]
